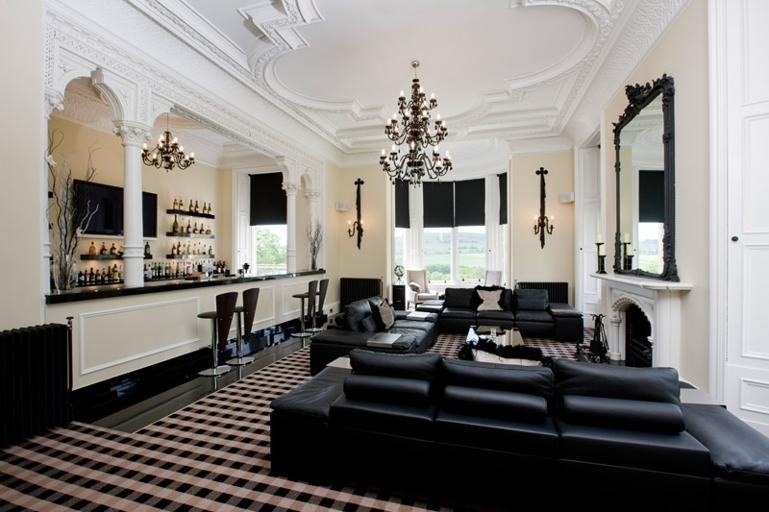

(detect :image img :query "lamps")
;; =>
[534,210,556,237]
[140,110,197,173]
[377,60,454,182]
[346,217,364,237]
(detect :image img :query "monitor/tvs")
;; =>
[74,178,159,241]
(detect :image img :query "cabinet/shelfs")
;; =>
[165,209,219,260]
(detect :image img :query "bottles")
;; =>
[77,264,124,286]
[89,241,96,254]
[144,261,191,278]
[172,214,211,235]
[193,260,227,274]
[110,243,116,254]
[172,241,213,255]
[145,240,150,255]
[172,197,212,215]
[100,241,107,254]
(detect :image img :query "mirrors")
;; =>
[613,72,682,283]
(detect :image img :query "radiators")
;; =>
[516,281,569,305]
[340,276,383,315]
[1,317,75,451]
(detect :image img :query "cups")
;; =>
[490,328,496,338]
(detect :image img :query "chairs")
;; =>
[305,278,330,332]
[226,288,264,365]
[483,269,504,287]
[194,290,239,376]
[406,268,438,309]
[290,280,319,339]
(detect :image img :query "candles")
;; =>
[622,231,632,243]
[598,243,606,255]
[626,244,634,255]
[596,231,603,243]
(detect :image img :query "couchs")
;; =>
[308,294,439,378]
[367,298,397,331]
[440,286,586,344]
[269,347,767,511]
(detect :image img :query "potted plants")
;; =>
[50,253,78,294]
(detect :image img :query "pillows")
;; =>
[471,286,509,313]
